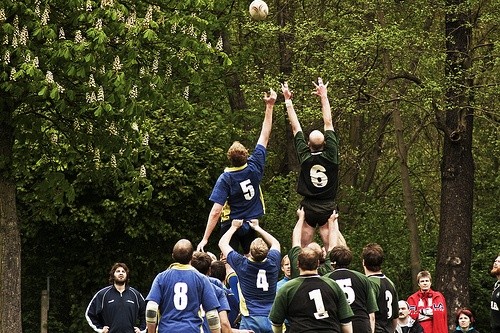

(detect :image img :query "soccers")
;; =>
[249,0,269,21]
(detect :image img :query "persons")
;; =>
[85,238,500,333]
[196,86,278,254]
[280,77,346,245]
